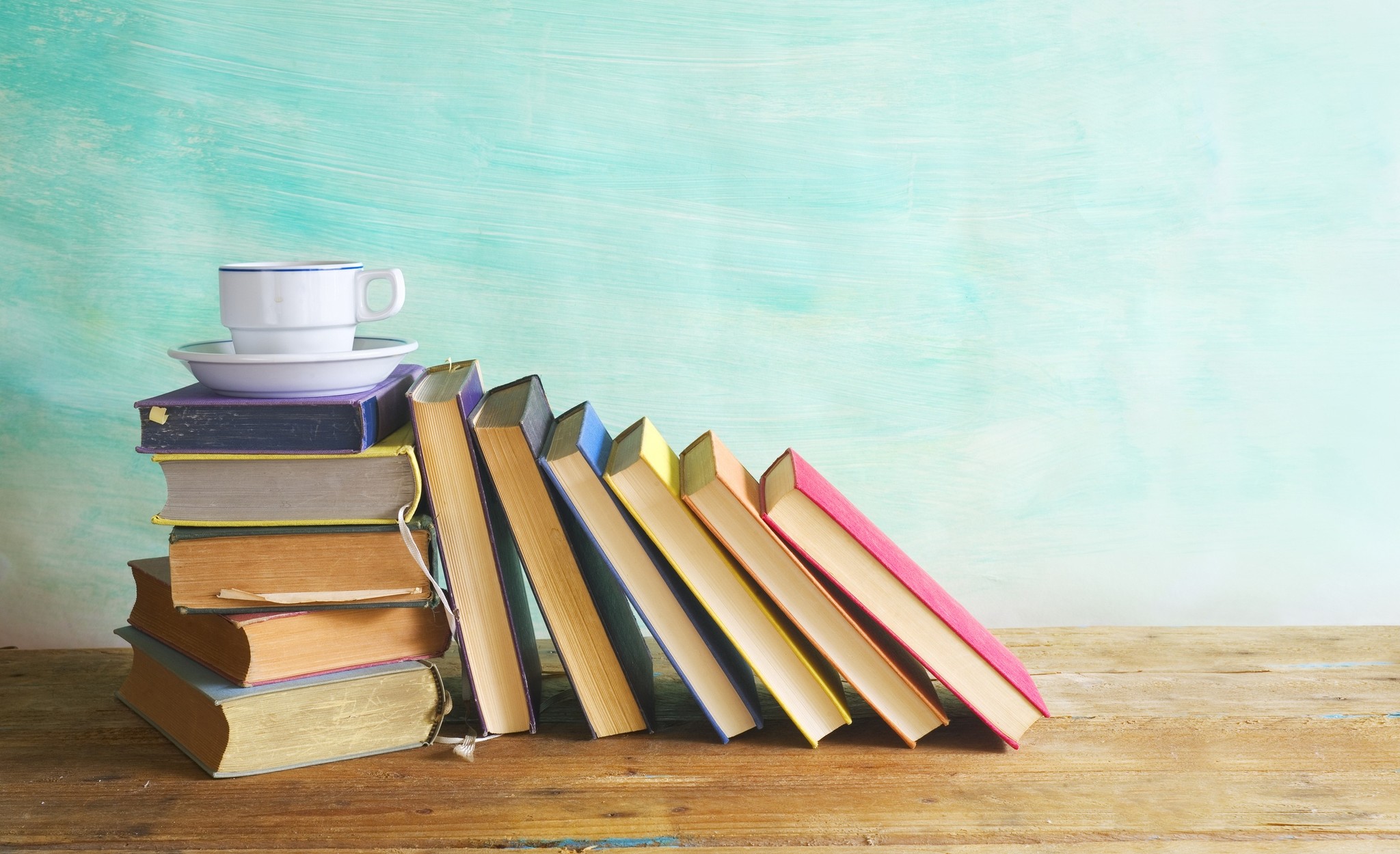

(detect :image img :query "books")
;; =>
[112,359,1054,779]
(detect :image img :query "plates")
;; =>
[167,334,420,399]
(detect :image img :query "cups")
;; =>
[217,258,406,355]
